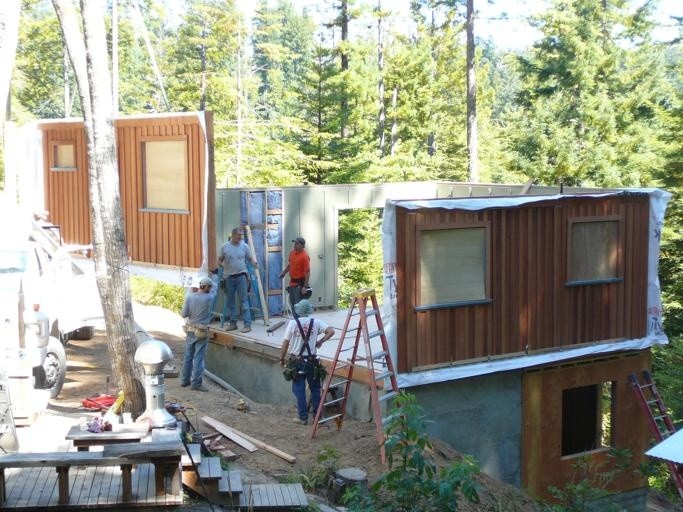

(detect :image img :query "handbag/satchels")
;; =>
[282,352,304,382]
[219,278,224,291]
[307,357,327,382]
[300,282,312,300]
[181,322,209,338]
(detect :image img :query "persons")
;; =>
[179,267,220,392]
[216,227,259,334]
[279,299,336,425]
[277,237,310,320]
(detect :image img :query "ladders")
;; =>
[308,289,410,465]
[630,369,683,491]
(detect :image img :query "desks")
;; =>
[65,422,150,470]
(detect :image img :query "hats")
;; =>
[199,276,215,287]
[294,299,313,316]
[292,237,305,248]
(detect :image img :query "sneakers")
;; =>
[224,319,252,333]
[292,416,329,425]
[180,382,209,392]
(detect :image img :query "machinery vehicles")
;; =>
[28,227,95,400]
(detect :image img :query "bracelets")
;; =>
[321,339,325,342]
[280,358,285,361]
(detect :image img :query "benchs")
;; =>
[0,451,180,508]
